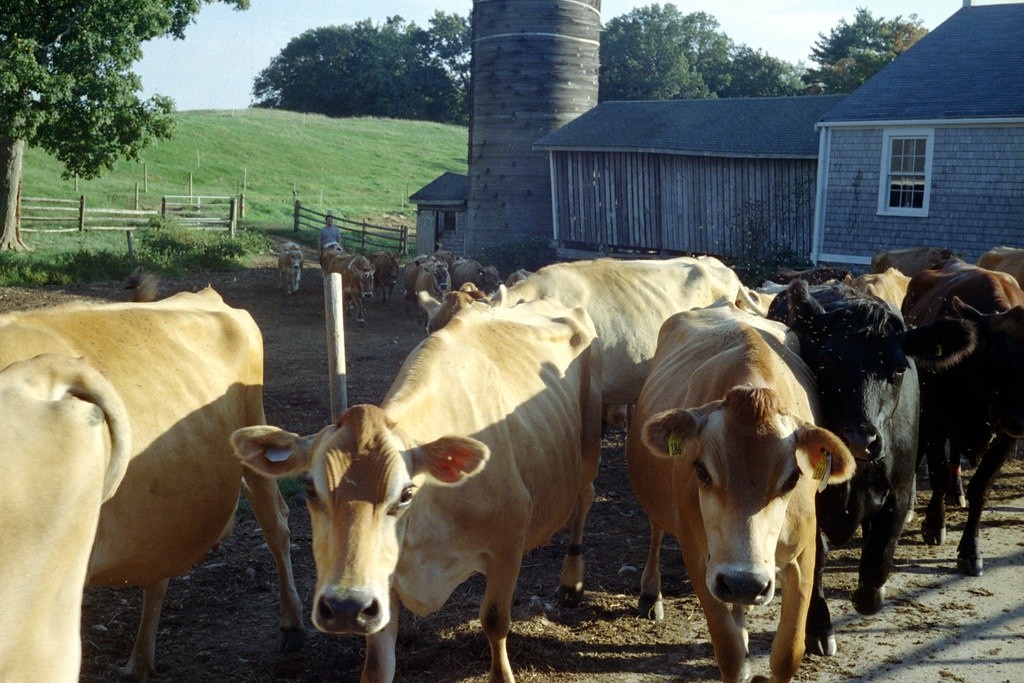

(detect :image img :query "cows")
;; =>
[0,240,1024,683]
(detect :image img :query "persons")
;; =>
[317,215,341,267]
